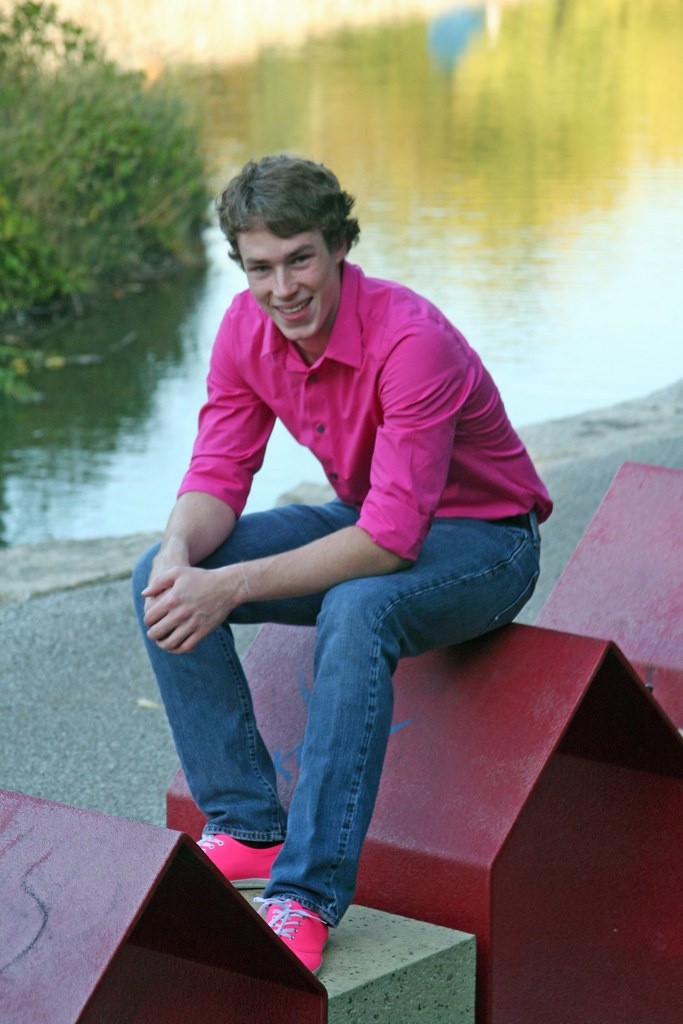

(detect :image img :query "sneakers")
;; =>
[196,834,284,888]
[253,897,330,975]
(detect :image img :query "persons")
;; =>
[132,156,555,978]
